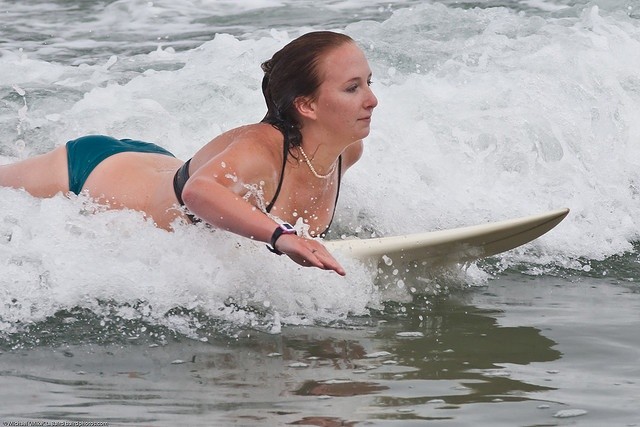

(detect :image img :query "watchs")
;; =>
[266,221,298,255]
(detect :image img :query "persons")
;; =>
[0,30,378,276]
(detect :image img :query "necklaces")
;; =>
[298,140,336,179]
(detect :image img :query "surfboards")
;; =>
[324,208,570,274]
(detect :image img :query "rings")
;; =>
[312,247,317,254]
